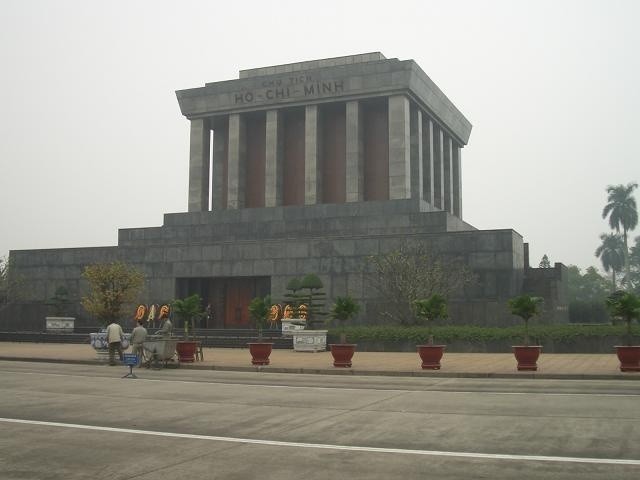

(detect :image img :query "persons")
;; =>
[152,313,173,337]
[130,320,148,368]
[105,319,126,366]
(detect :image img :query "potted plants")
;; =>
[44,286,76,332]
[324,296,359,366]
[279,277,309,335]
[169,294,206,361]
[604,288,640,371]
[246,294,277,365]
[413,292,450,370]
[506,296,544,371]
[291,275,329,351]
